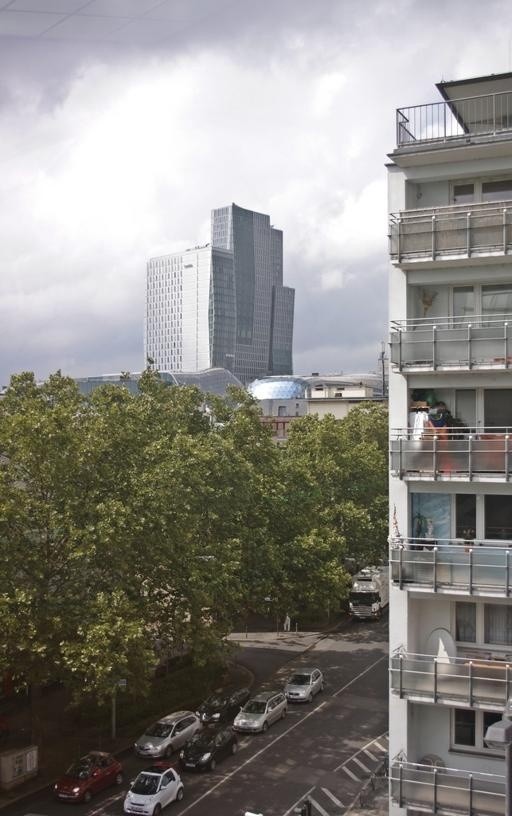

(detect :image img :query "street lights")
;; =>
[377,339,389,397]
[484,699,512,816]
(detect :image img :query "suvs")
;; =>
[134,710,203,761]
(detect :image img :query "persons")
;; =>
[284,614,290,632]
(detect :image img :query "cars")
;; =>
[231,691,288,735]
[177,725,238,773]
[283,667,326,704]
[123,765,184,816]
[53,750,121,803]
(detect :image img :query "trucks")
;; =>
[348,565,389,622]
